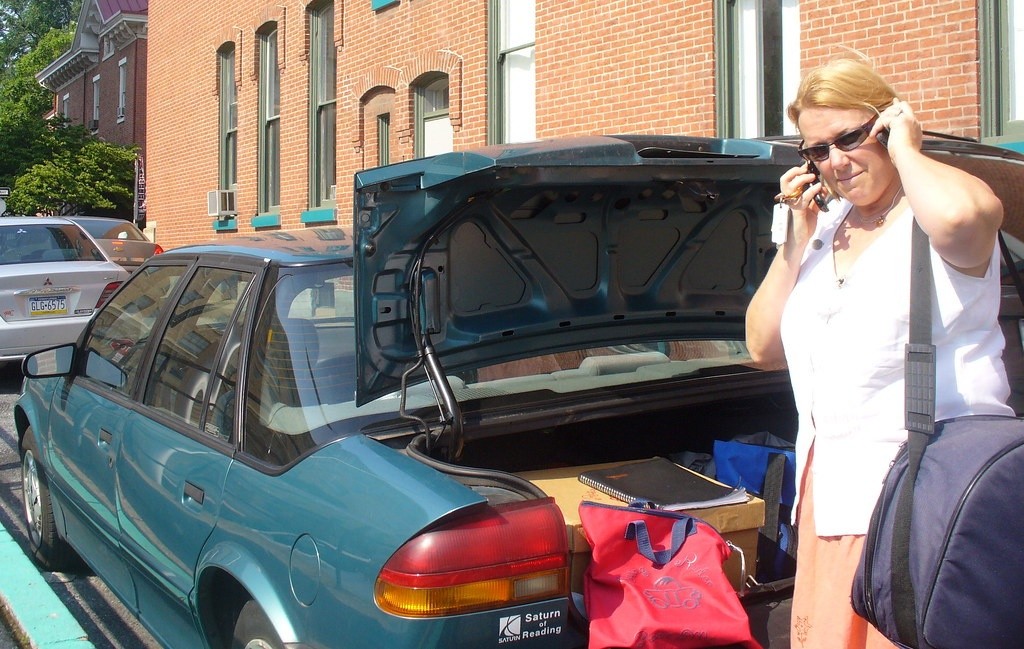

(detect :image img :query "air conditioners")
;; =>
[207,190,236,216]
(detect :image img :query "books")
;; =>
[578,457,750,513]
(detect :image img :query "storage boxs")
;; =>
[510,458,765,592]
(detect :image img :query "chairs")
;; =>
[279,317,319,367]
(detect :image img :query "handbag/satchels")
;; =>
[852,413,1024,648]
[577,497,762,649]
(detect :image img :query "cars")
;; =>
[13,130,1024,649]
[66,216,171,297]
[0,217,131,380]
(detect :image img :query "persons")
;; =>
[745,59,1018,649]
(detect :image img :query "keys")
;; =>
[770,203,791,245]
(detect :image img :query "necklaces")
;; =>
[853,186,902,225]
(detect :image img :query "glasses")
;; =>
[798,114,879,161]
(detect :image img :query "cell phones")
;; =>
[807,159,829,213]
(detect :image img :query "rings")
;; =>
[895,108,903,115]
